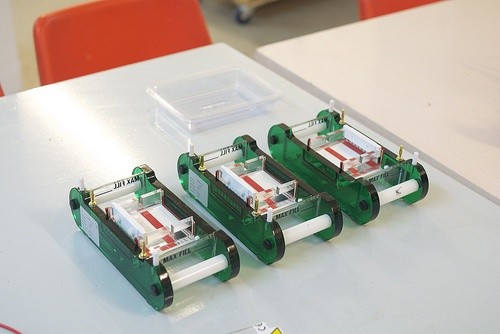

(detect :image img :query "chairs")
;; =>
[30,0,213,88]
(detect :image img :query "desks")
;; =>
[252,0,500,208]
[0,40,500,334]
